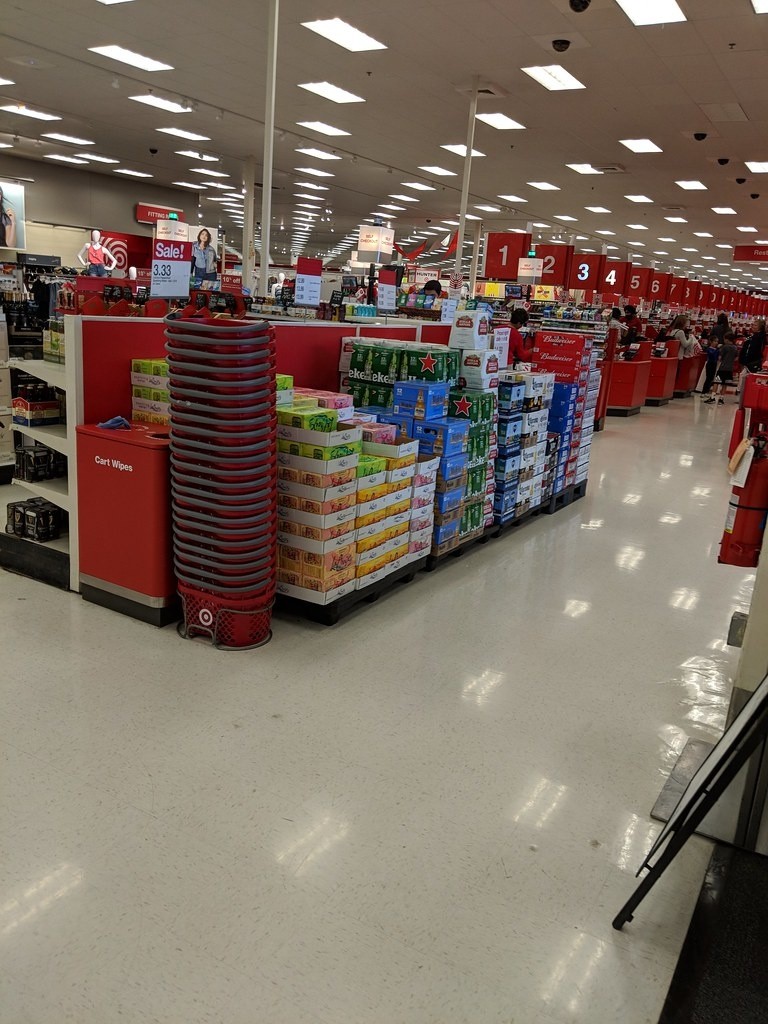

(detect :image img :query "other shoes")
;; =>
[704,397,716,403]
[717,399,724,406]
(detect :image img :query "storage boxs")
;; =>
[274,307,604,606]
[128,359,174,428]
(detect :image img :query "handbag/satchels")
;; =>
[684,334,702,358]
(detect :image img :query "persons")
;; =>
[709,313,733,344]
[271,272,286,297]
[665,315,679,340]
[191,229,217,281]
[700,335,723,401]
[704,331,739,405]
[606,304,642,338]
[423,280,442,298]
[670,314,693,401]
[0,186,17,248]
[76,230,117,277]
[491,308,533,370]
[738,319,766,409]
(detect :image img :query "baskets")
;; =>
[161,309,278,646]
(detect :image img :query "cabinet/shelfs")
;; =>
[485,310,608,362]
[0,315,453,628]
